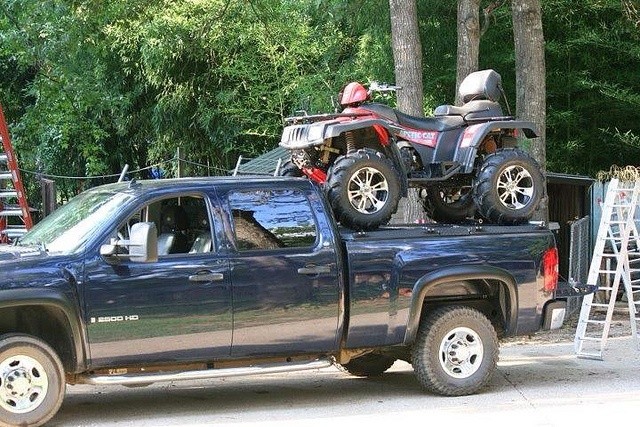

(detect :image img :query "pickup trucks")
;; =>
[0,176,564,426]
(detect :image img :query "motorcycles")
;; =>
[279,69,546,229]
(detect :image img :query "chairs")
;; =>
[155,205,188,256]
[189,210,214,254]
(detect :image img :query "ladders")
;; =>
[573,177,640,362]
[0,106,34,243]
[597,191,640,313]
